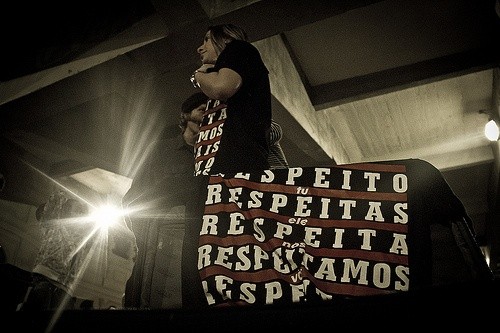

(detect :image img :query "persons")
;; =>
[180,92,289,168]
[180,24,273,176]
[15,176,139,312]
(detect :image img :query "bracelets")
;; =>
[189,70,205,89]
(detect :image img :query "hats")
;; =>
[180,92,208,113]
